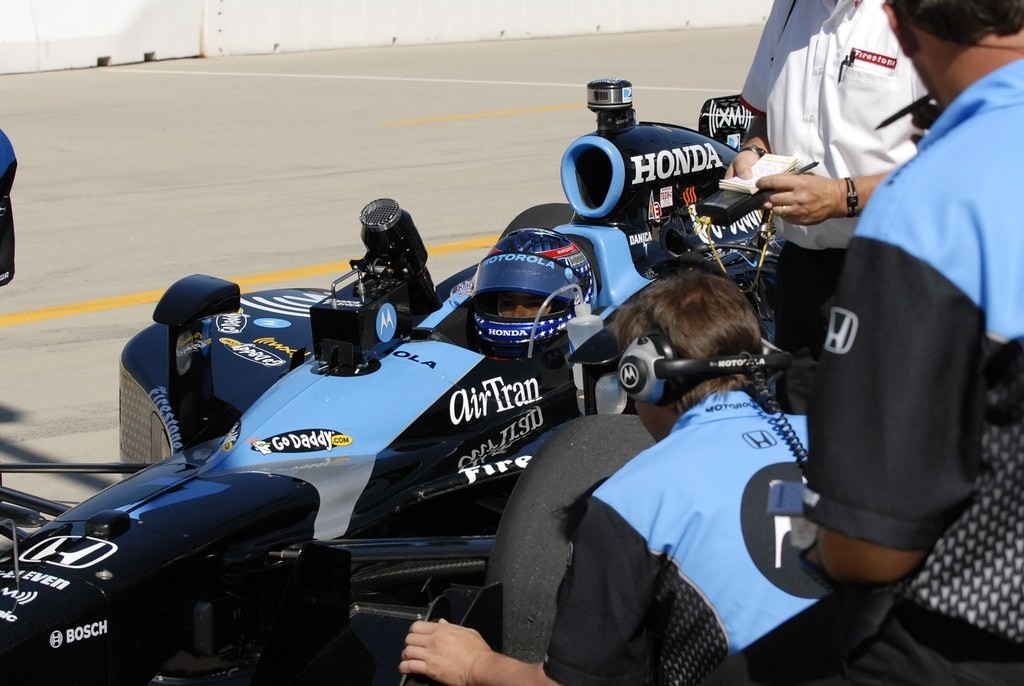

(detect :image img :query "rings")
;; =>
[781,205,786,219]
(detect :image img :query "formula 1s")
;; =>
[0,79,778,686]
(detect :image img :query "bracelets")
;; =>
[742,146,766,157]
[845,177,858,218]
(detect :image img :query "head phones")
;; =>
[618,334,794,406]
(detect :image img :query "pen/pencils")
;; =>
[876,94,932,130]
[792,161,819,175]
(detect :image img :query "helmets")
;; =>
[473,228,597,359]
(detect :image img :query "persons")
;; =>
[804,0,1024,686]
[721,0,945,364]
[469,227,593,363]
[0,131,18,286]
[397,268,863,685]
